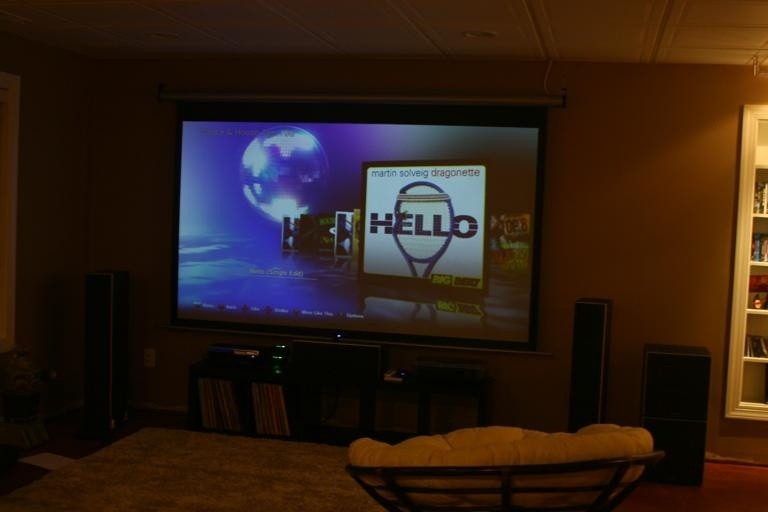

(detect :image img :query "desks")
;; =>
[1,427,390,512]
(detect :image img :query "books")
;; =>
[199,378,288,433]
[745,179,768,357]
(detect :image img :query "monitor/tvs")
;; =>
[170,112,548,352]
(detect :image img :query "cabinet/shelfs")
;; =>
[724,104,768,423]
[186,357,488,446]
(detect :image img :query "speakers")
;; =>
[640,343,712,421]
[287,339,382,384]
[641,415,706,486]
[79,270,129,439]
[567,297,613,434]
